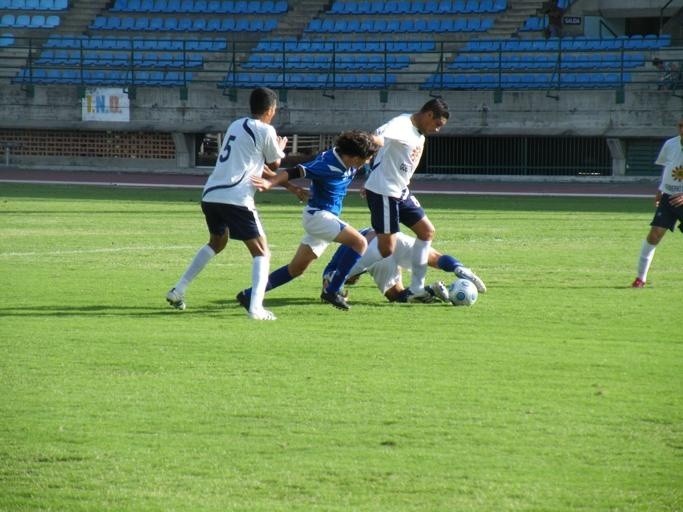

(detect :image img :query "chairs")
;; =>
[0,0,683,89]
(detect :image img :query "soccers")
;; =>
[447,279,477,307]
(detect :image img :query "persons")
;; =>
[322,226,487,303]
[632,114,683,287]
[236,129,371,315]
[163,86,309,320]
[652,56,678,88]
[324,94,454,302]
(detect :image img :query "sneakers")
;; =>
[236,290,275,320]
[409,266,486,302]
[166,288,185,310]
[321,289,350,310]
[633,278,644,287]
